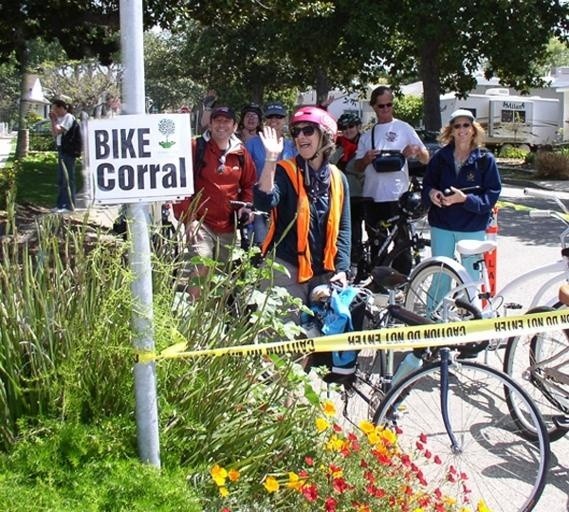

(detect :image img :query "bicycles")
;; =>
[152,201,179,292]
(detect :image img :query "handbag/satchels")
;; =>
[372,148,407,174]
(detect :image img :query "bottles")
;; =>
[390,346,426,391]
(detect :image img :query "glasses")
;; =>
[289,124,318,139]
[341,123,357,130]
[452,123,472,129]
[215,154,228,173]
[374,103,394,109]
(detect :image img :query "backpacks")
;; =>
[60,119,83,159]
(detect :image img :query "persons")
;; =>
[82,85,431,377]
[47,99,84,214]
[421,110,503,349]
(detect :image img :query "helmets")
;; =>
[241,102,263,116]
[289,104,339,136]
[336,112,363,129]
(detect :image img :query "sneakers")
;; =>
[49,204,76,214]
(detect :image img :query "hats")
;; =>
[263,100,288,117]
[211,106,236,119]
[447,109,475,124]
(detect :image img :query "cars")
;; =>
[27,118,52,133]
